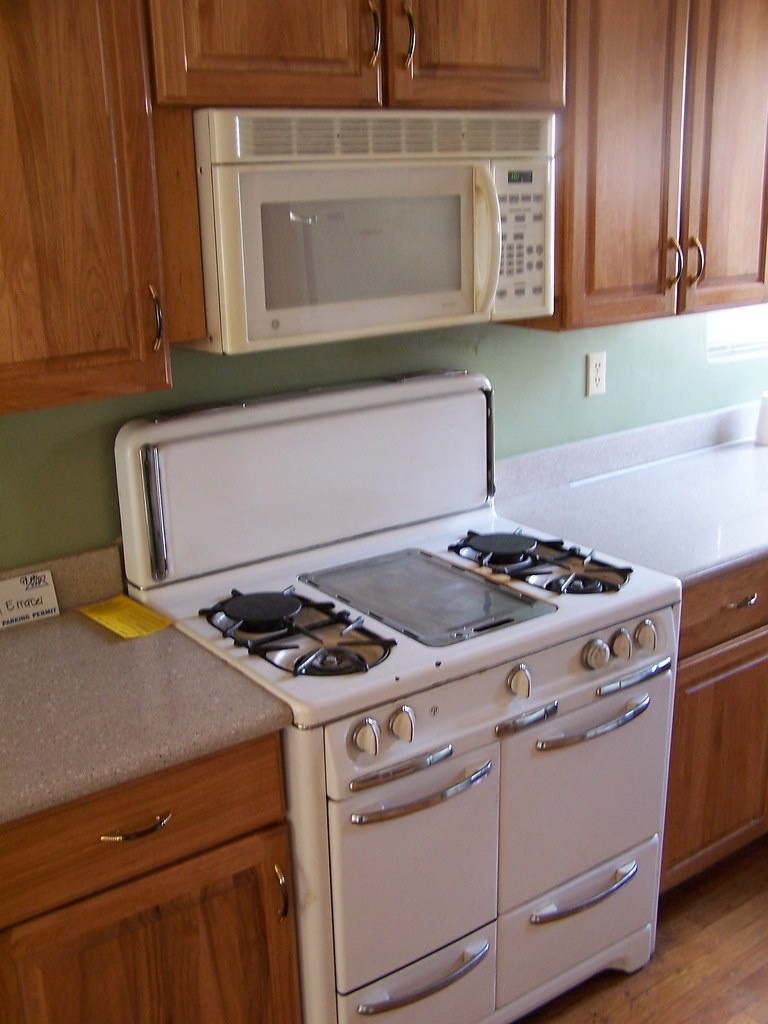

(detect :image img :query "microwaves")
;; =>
[192,105,560,352]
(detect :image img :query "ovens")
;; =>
[284,658,683,1024]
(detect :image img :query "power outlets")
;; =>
[587,351,607,397]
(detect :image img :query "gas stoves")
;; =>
[113,367,683,730]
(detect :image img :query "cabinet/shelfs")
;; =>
[660,558,768,893]
[1,733,305,1023]
[0,0,767,418]
